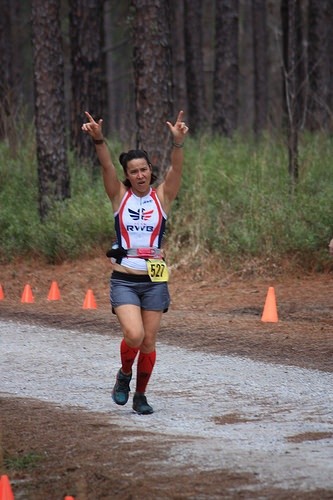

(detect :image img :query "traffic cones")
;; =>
[64,495,76,500]
[0,283,6,302]
[261,287,279,323]
[47,280,63,301]
[81,289,97,310]
[19,284,36,304]
[0,474,16,500]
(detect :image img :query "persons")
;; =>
[81,111,189,415]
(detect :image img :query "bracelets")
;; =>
[94,140,104,144]
[172,141,184,148]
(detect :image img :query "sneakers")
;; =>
[112,368,132,405]
[132,395,153,415]
